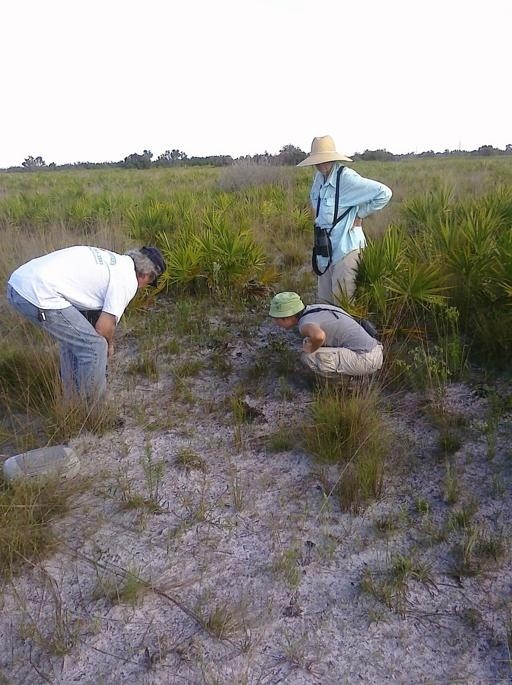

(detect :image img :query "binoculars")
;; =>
[314,227,329,257]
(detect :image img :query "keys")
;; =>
[38,309,46,322]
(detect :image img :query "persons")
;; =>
[269,292,384,394]
[6,246,166,426]
[297,135,392,313]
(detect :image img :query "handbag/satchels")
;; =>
[351,315,377,337]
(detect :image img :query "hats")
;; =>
[268,292,305,318]
[139,246,166,288]
[297,135,353,166]
